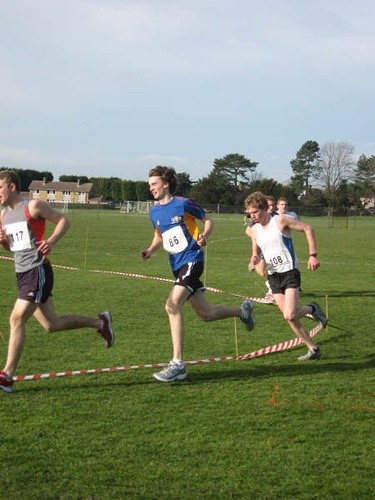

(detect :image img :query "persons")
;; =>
[243,191,329,361]
[140,165,255,381]
[0,170,115,391]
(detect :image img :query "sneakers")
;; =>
[298,346,321,360]
[153,360,187,382]
[96,311,114,348]
[239,300,255,331]
[0,370,14,393]
[308,301,327,327]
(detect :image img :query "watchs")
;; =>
[308,253,317,257]
